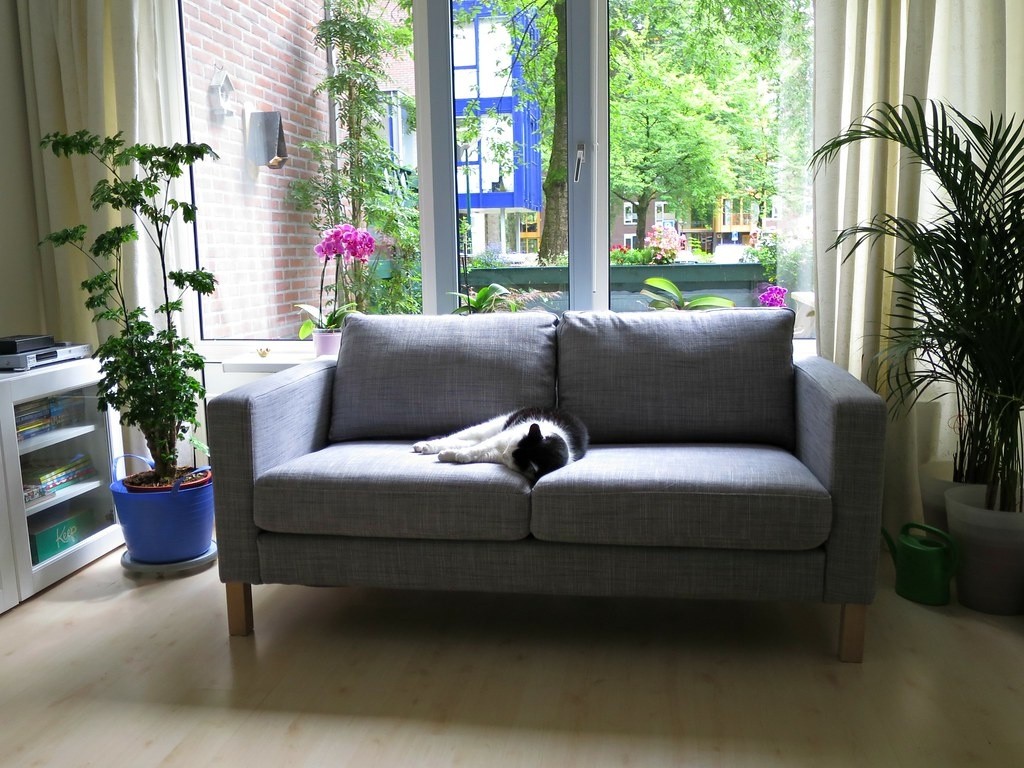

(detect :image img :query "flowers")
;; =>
[609,243,632,265]
[644,221,685,265]
[291,220,377,339]
[758,285,788,308]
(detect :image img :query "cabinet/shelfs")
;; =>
[0,353,125,615]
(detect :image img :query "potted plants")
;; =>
[36,129,216,566]
[805,92,1024,618]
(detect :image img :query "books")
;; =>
[23,454,99,503]
[14,390,85,441]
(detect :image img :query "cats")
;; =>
[413,401,590,489]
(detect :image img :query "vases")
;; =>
[313,328,342,358]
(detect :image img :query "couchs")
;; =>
[206,309,886,663]
